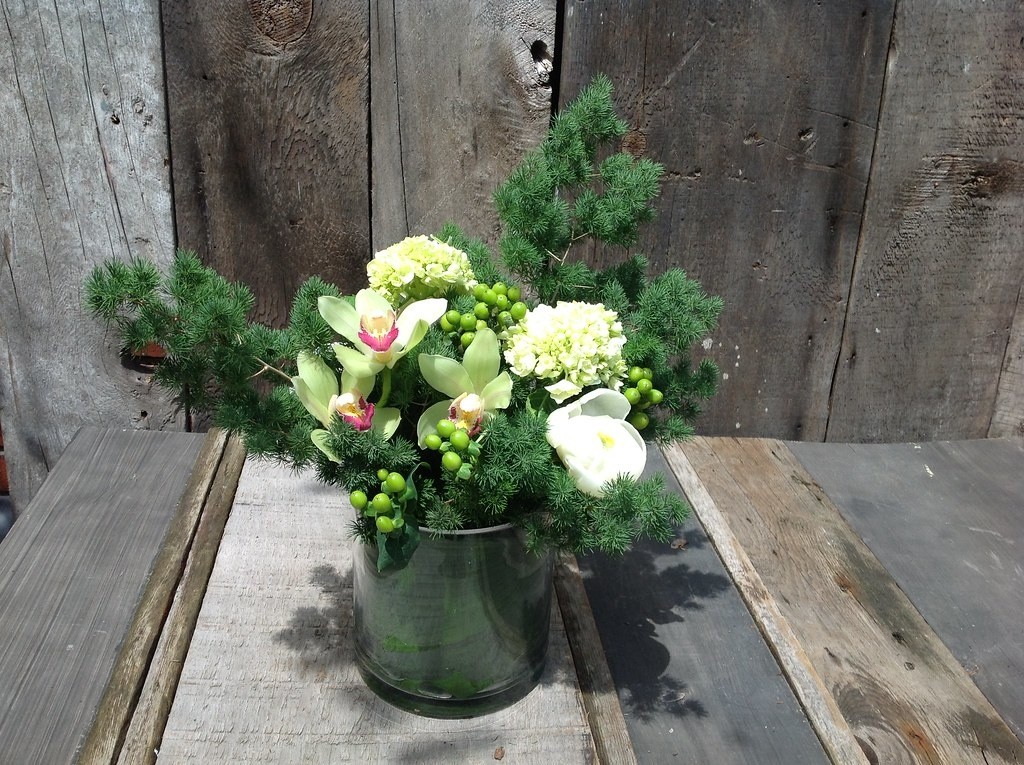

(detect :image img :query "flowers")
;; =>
[82,70,724,577]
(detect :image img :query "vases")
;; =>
[344,519,564,727]
